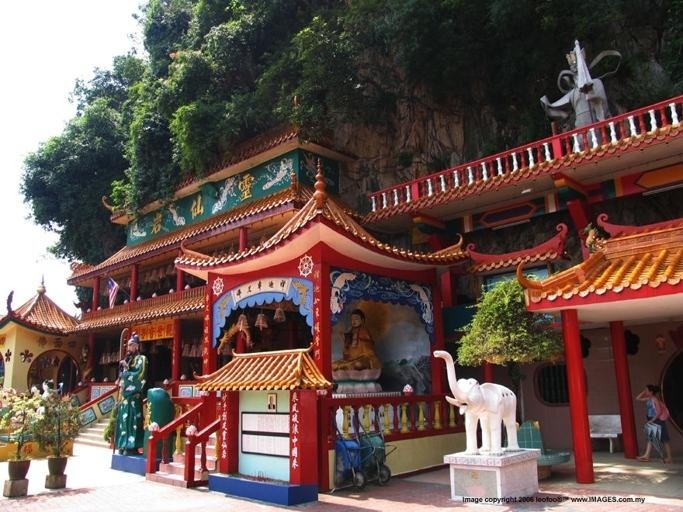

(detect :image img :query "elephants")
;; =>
[433,350,520,457]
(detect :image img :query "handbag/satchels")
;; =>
[653,399,672,422]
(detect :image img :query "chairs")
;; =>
[586,414,622,454]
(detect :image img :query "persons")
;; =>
[115,335,149,457]
[636,385,672,464]
[541,45,609,154]
[331,310,381,371]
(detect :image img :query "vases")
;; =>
[4,456,71,497]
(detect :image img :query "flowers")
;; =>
[0,390,79,459]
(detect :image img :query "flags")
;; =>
[107,277,119,308]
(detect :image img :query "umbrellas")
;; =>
[642,421,666,463]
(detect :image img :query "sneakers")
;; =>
[637,456,649,461]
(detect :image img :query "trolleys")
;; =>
[329,408,397,491]
